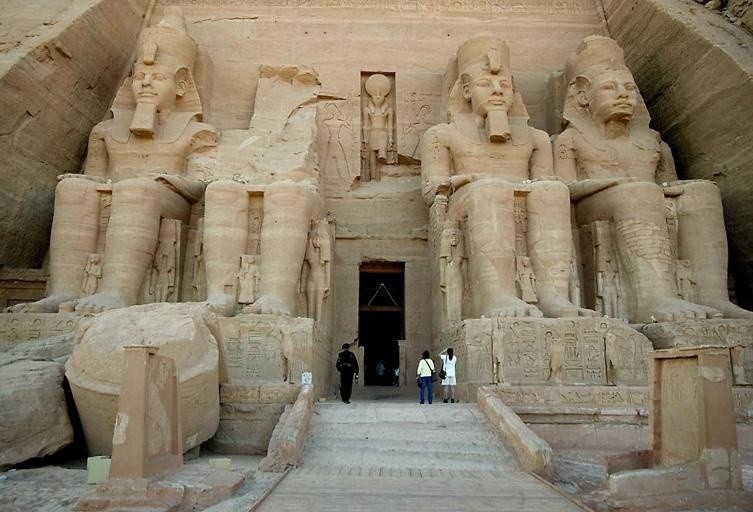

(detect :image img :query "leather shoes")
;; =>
[420,401,432,404]
[443,399,454,403]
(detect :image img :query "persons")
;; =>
[203,180,310,322]
[415,350,435,404]
[437,348,458,403]
[300,221,331,323]
[3,5,219,313]
[362,90,394,180]
[551,35,753,321]
[421,35,602,319]
[336,343,359,404]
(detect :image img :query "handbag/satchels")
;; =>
[431,370,438,382]
[439,369,446,379]
